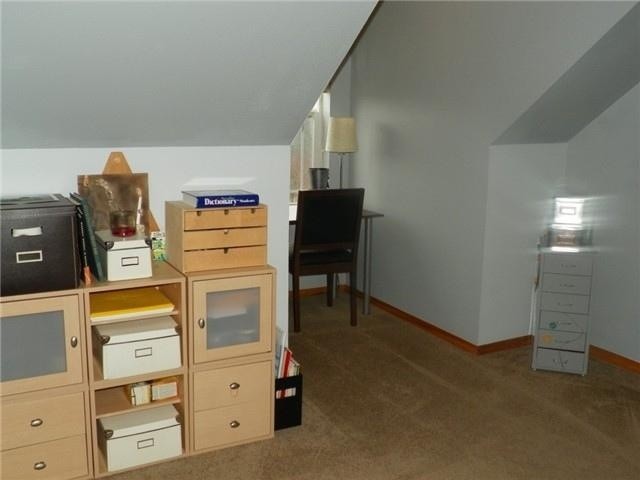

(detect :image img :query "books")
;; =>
[274,324,300,399]
[181,190,261,209]
[65,196,92,285]
[69,192,104,281]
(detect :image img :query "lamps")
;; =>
[325,118,359,188]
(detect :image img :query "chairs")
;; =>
[289,188,365,332]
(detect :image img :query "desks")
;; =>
[290,204,385,315]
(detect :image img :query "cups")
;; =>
[110,211,139,236]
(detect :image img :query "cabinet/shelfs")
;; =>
[0,288,93,480]
[166,204,268,273]
[186,264,275,455]
[83,262,185,479]
[531,251,592,376]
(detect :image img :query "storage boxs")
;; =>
[92,333,181,379]
[0,202,76,293]
[275,374,302,431]
[96,241,152,281]
[95,423,183,471]
